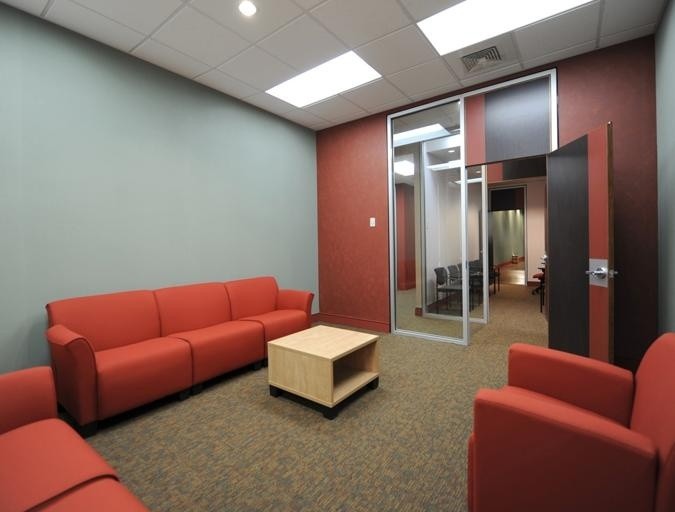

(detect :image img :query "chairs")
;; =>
[464,333,675,511]
[434,257,500,316]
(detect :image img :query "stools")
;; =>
[532,255,545,313]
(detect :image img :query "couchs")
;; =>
[0,365,154,512]
[44,277,314,436]
